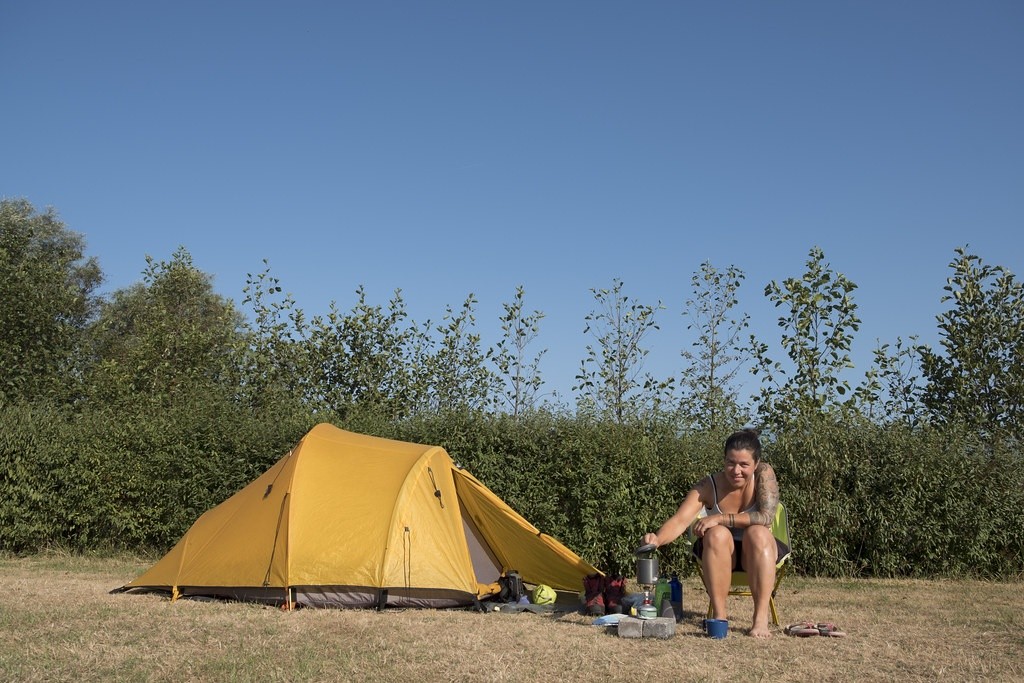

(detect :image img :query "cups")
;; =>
[636,559,659,584]
[706,619,728,639]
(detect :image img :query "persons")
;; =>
[640,428,790,639]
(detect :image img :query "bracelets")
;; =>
[723,512,736,529]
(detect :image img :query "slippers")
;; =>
[783,620,846,638]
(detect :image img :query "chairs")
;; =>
[691,500,789,630]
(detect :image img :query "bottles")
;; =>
[668,571,683,623]
[643,586,653,606]
[655,578,671,617]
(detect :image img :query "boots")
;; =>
[583,572,606,617]
[604,573,628,614]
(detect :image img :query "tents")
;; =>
[121,423,629,615]
